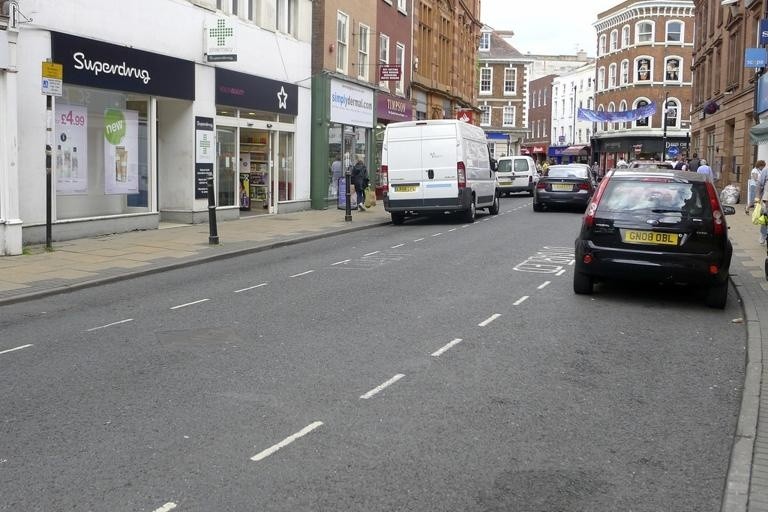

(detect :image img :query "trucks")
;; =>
[380,119,500,224]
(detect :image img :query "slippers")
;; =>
[745,206,750,216]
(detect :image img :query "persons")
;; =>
[352,161,371,214]
[532,152,715,190]
[758,165,767,246]
[747,159,766,215]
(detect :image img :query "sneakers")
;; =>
[759,235,767,244]
[357,201,365,211]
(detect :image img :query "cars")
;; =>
[533,165,594,213]
[574,168,733,310]
[565,162,596,167]
[620,161,673,171]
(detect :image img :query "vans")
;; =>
[492,154,540,199]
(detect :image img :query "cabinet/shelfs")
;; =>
[219,139,268,203]
